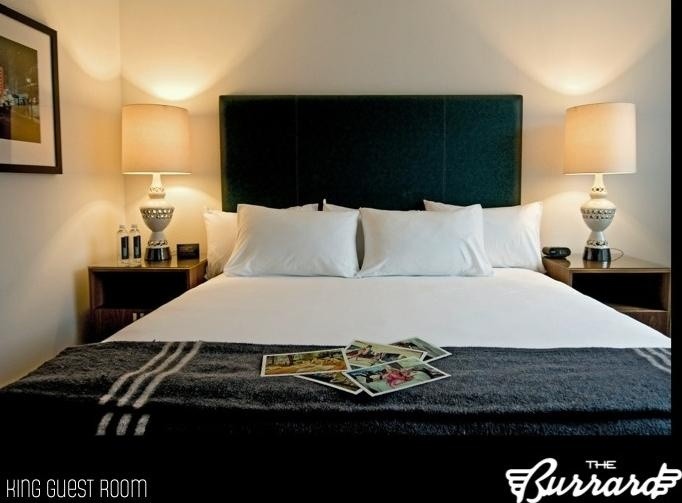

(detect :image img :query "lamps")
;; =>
[120,102,194,262]
[563,102,637,262]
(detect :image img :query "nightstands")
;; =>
[86,255,207,343]
[542,253,671,337]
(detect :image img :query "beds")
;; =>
[1,266,672,503]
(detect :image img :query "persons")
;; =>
[323,343,413,387]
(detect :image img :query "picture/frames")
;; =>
[0,3,63,175]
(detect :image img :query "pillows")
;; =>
[201,198,548,279]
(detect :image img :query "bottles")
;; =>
[116,223,143,268]
